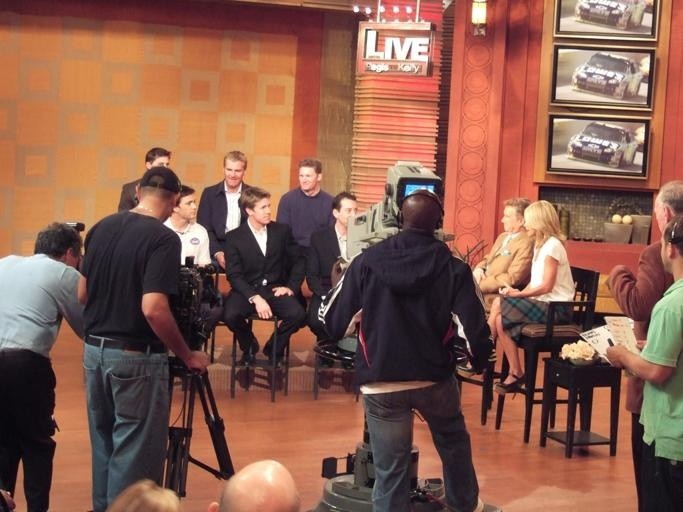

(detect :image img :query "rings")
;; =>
[7,491,11,496]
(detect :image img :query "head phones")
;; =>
[396,192,444,230]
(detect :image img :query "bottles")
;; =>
[558,210,570,240]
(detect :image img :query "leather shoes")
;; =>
[456,364,481,375]
[311,340,354,371]
[261,343,285,369]
[238,339,260,366]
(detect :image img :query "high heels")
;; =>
[497,371,525,400]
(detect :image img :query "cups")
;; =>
[602,222,633,244]
[630,214,651,245]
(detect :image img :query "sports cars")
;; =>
[572,50,643,100]
[574,0,645,30]
[567,122,638,168]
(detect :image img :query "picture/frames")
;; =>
[552,0,662,44]
[548,42,658,113]
[545,110,653,181]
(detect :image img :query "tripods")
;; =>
[158,357,235,502]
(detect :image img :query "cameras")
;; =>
[67,222,84,273]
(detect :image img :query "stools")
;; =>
[539,356,622,457]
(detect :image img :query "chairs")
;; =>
[496,266,601,444]
[206,272,240,364]
[230,313,290,403]
[455,337,529,425]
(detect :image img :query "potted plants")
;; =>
[608,195,652,246]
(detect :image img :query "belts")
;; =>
[83,335,167,354]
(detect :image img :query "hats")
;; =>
[138,166,185,194]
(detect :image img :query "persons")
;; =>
[318,189,497,512]
[105,478,180,512]
[0,489,16,512]
[208,459,301,512]
[456,200,575,392]
[77,167,211,512]
[472,198,536,320]
[0,223,85,512]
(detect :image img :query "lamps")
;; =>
[471,0,488,37]
